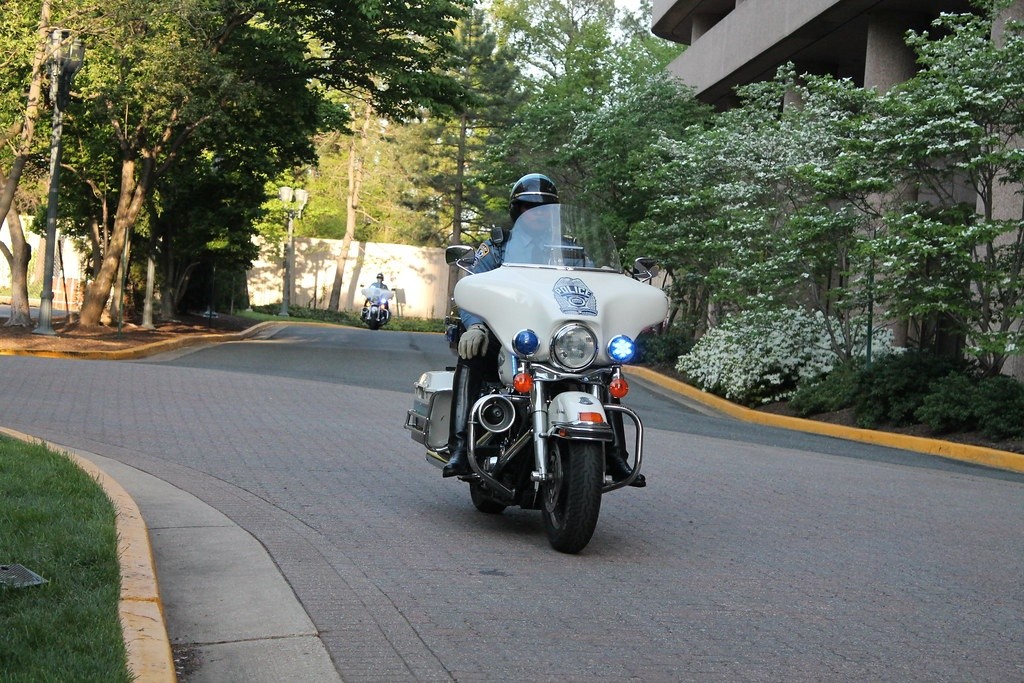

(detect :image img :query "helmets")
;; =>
[508,173,559,222]
[377,274,384,281]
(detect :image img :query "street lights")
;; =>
[278,186,309,316]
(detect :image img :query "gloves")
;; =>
[458,324,490,359]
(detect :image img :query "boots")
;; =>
[442,361,492,477]
[604,386,646,487]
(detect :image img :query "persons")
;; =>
[442,172,647,490]
[363,273,390,313]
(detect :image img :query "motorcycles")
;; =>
[402,204,668,551]
[360,284,397,329]
